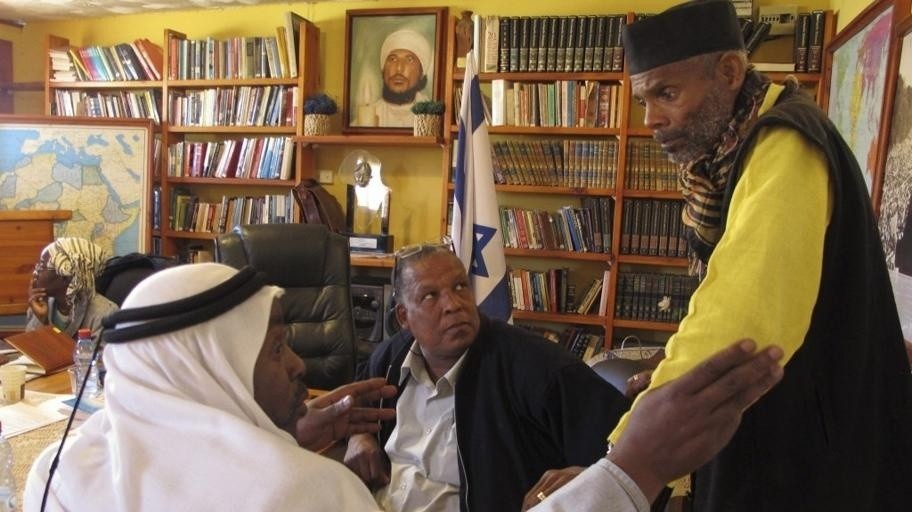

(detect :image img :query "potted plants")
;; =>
[303,91,337,136]
[410,97,446,136]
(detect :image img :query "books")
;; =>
[741,10,824,73]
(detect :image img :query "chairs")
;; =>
[94,253,180,309]
[585,344,666,397]
[215,221,357,390]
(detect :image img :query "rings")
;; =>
[634,374,639,380]
[537,492,546,501]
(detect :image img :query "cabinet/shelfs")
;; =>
[439,9,831,354]
[44,19,321,264]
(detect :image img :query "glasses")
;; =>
[390,234,457,299]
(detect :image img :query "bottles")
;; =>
[73,327,100,405]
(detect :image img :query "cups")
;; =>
[0,364,27,402]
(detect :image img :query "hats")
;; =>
[620,0,746,77]
[379,29,434,74]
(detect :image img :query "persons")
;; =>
[350,29,431,128]
[22,262,785,512]
[24,238,121,348]
[519,0,912,512]
[342,244,674,512]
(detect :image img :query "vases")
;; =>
[453,10,474,74]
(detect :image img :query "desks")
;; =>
[348,249,398,344]
[0,356,350,512]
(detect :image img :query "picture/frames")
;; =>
[340,4,450,136]
[0,114,156,259]
[817,0,904,228]
